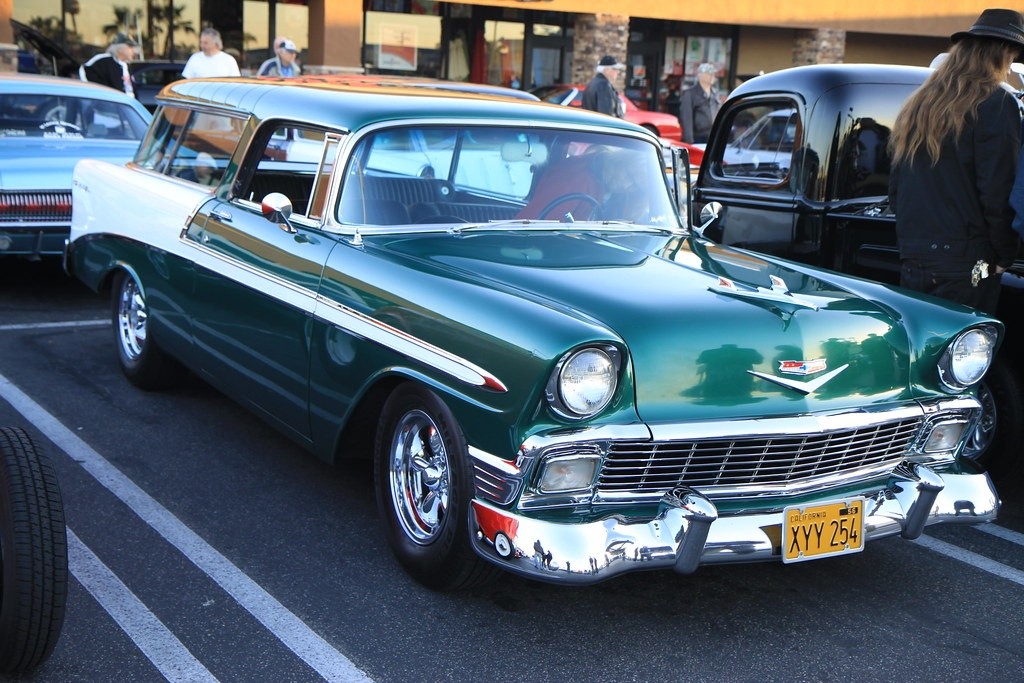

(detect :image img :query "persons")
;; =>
[181,29,241,132]
[515,149,652,221]
[176,152,219,183]
[887,9,1024,318]
[679,63,722,144]
[582,55,625,120]
[256,39,301,77]
[79,30,138,137]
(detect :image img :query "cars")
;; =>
[0,71,217,269]
[566,134,728,203]
[526,83,683,141]
[70,75,1002,588]
[692,56,1024,526]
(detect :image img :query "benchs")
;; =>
[237,169,522,222]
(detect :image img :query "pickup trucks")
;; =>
[692,108,798,183]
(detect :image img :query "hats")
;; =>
[280,41,300,55]
[111,32,138,46]
[197,151,218,172]
[952,9,1024,65]
[599,56,627,71]
[697,64,716,74]
